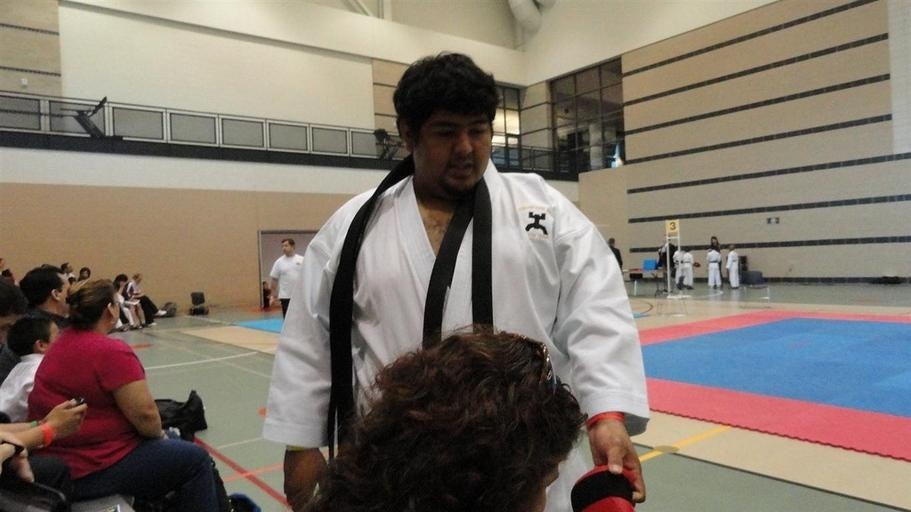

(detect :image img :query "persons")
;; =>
[263,281,274,308]
[659,236,739,291]
[607,237,623,268]
[315,331,588,512]
[263,52,649,510]
[1,263,259,512]
[263,238,303,318]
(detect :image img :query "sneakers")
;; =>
[117,309,167,332]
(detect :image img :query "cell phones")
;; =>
[70,395,85,408]
